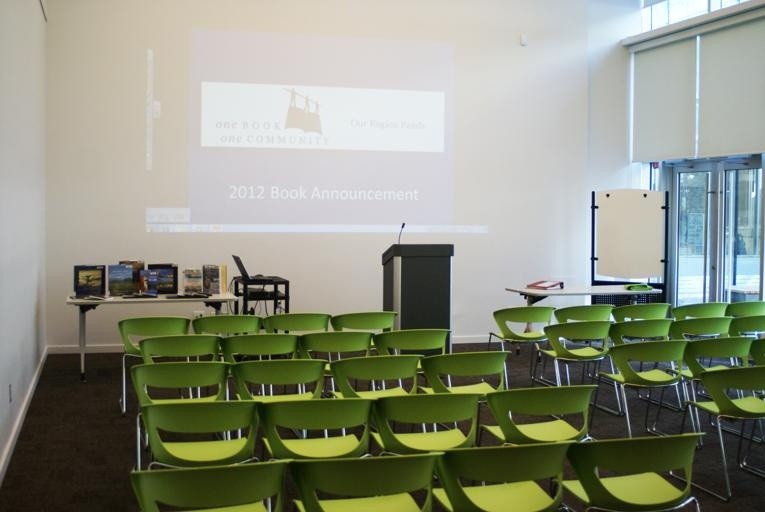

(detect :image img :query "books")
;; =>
[108,260,145,295]
[203,263,228,295]
[183,268,203,295]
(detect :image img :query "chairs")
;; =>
[128,360,229,399]
[116,317,189,414]
[140,332,220,361]
[371,394,478,452]
[560,430,703,512]
[728,316,765,366]
[225,331,298,360]
[670,366,765,500]
[373,327,452,355]
[137,399,254,462]
[192,314,260,400]
[749,339,765,369]
[436,440,576,512]
[608,318,679,415]
[529,322,612,420]
[591,341,687,437]
[665,337,755,449]
[130,460,287,511]
[302,330,376,360]
[612,304,673,397]
[230,358,327,396]
[259,394,372,457]
[726,300,764,316]
[479,386,596,444]
[670,317,735,414]
[263,314,331,404]
[291,454,442,511]
[671,302,729,367]
[332,354,419,396]
[555,304,616,402]
[331,309,399,392]
[486,307,555,390]
[422,349,509,430]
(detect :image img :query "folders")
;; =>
[526,280,565,290]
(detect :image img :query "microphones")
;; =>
[398,222,405,244]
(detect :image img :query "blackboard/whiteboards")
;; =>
[588,188,669,289]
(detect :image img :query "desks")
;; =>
[66,290,237,377]
[382,244,453,356]
[506,284,662,336]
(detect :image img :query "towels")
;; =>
[625,282,652,292]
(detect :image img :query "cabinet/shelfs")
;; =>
[233,275,289,314]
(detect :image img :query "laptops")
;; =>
[231,254,272,282]
